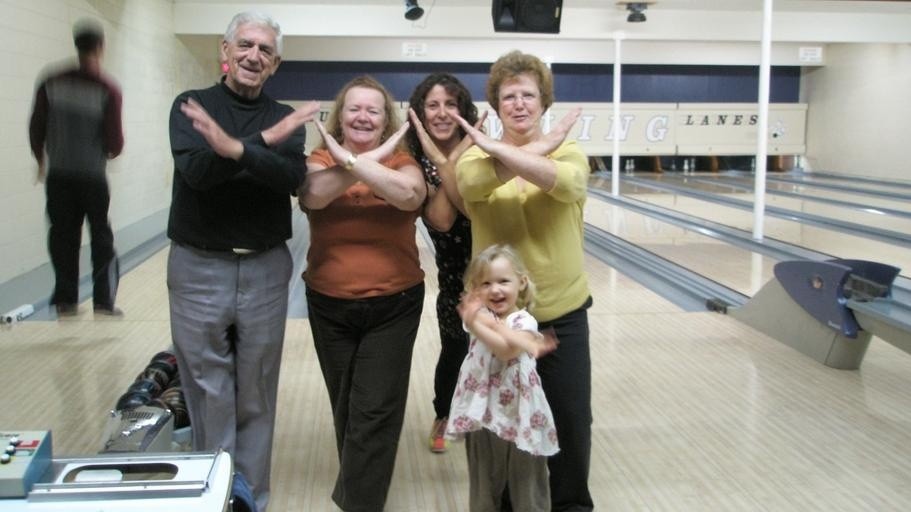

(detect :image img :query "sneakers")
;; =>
[56,302,78,315]
[95,307,125,322]
[429,416,453,453]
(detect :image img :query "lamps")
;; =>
[403,0,423,20]
[627,2,649,21]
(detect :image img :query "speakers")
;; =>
[492,0,562,33]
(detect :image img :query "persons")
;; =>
[443,242,564,511]
[163,11,327,510]
[402,71,490,454]
[292,72,433,510]
[28,16,127,318]
[453,46,599,512]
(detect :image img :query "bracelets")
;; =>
[339,151,360,172]
